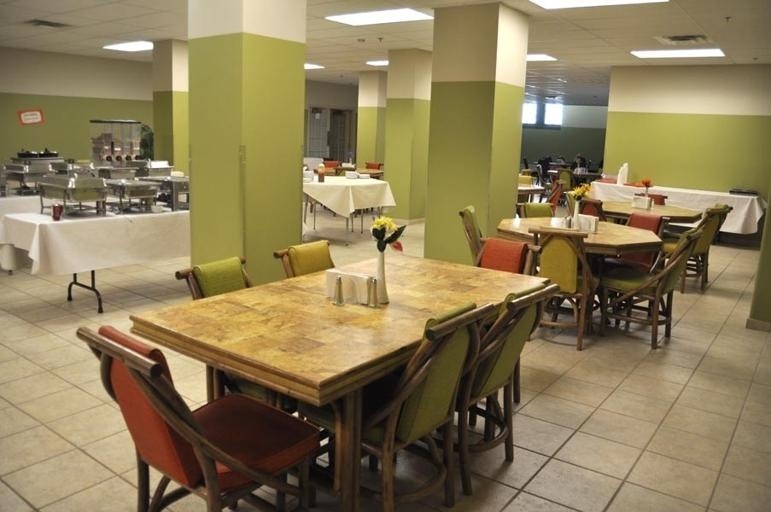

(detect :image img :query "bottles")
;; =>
[333,276,345,305]
[318,164,325,182]
[368,279,378,308]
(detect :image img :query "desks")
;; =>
[592,183,761,246]
[0,196,191,313]
